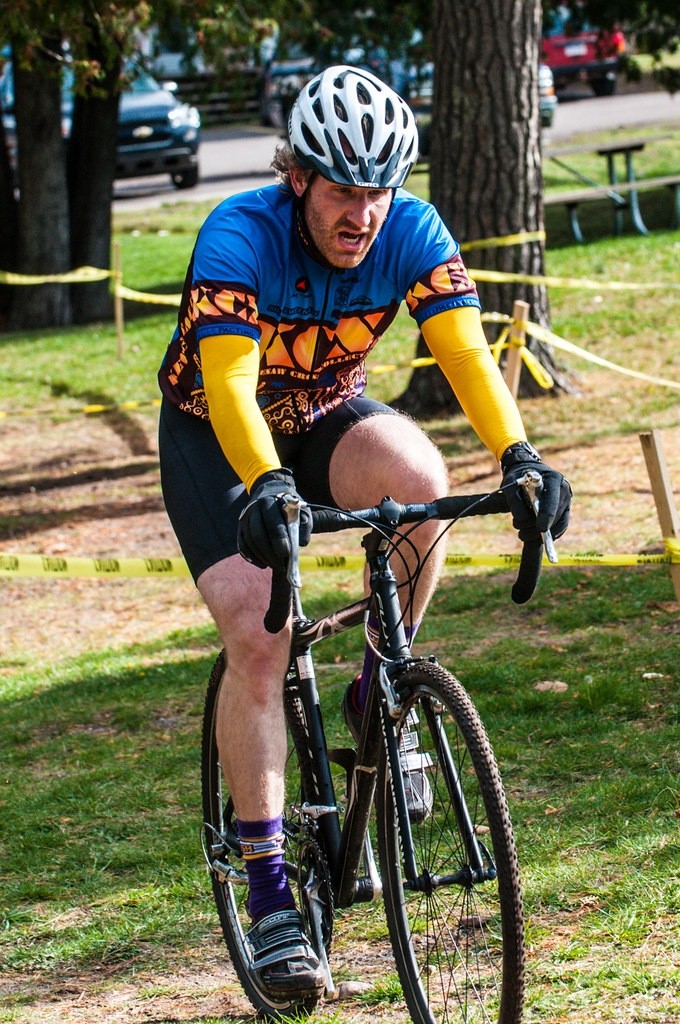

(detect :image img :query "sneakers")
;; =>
[244,910,323,1000]
[341,674,434,826]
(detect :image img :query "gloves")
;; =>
[499,441,574,544]
[237,468,314,570]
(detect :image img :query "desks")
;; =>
[541,138,653,241]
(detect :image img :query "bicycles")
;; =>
[198,465,557,1023]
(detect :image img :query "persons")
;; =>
[159,65,573,994]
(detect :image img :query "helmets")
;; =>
[287,64,419,190]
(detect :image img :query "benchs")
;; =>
[540,174,680,247]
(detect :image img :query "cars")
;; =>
[264,26,557,155]
[3,59,202,187]
[539,4,626,96]
